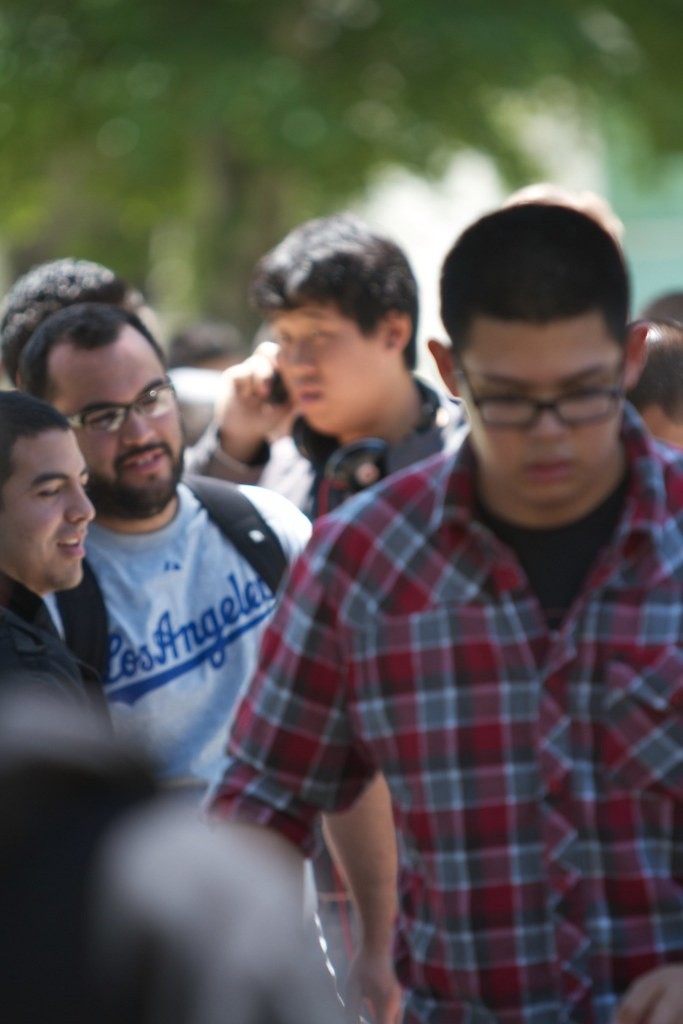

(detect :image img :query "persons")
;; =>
[626,293,683,445]
[197,199,683,1024]
[0,389,192,1024]
[184,214,466,528]
[0,258,165,387]
[15,302,314,782]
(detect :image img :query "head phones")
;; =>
[291,377,442,494]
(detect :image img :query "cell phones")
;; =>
[264,370,287,405]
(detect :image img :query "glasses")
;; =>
[457,353,625,427]
[67,376,176,433]
